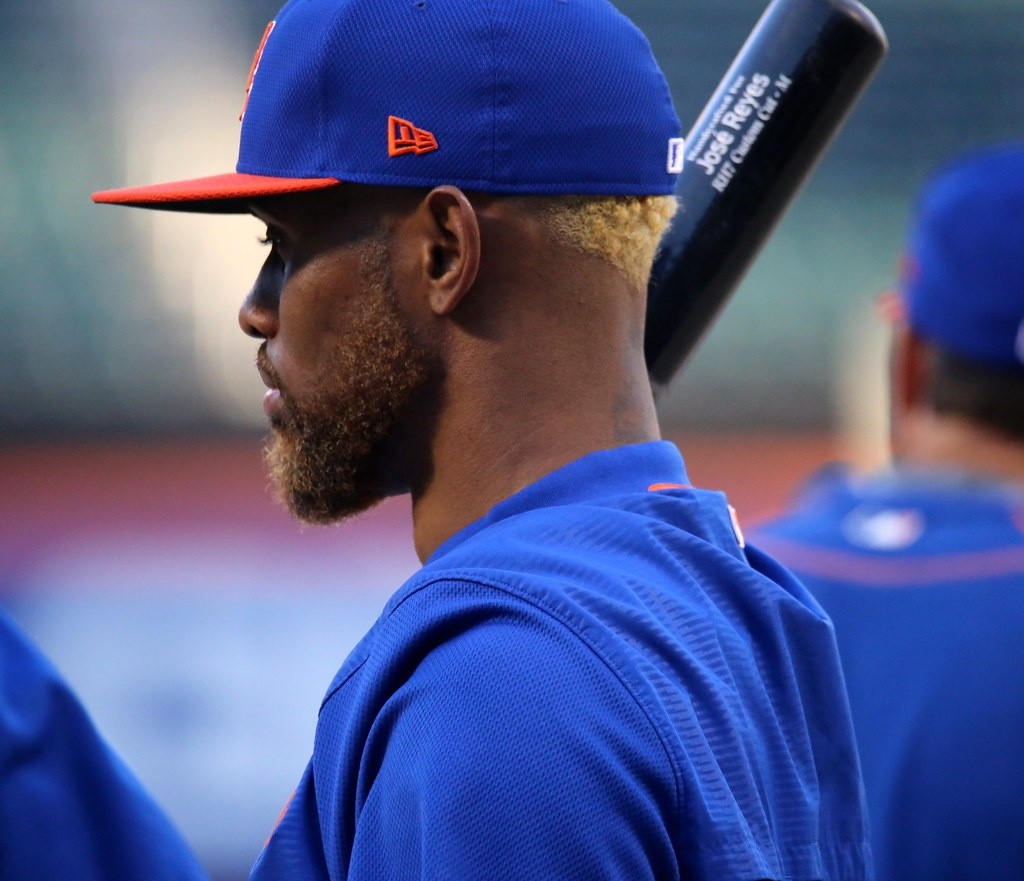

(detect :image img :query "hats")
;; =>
[899,148,1024,359]
[90,0,682,215]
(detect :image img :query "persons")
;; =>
[740,129,1024,881]
[93,1,876,881]
[0,609,207,881]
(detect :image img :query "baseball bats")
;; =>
[645,0,890,407]
